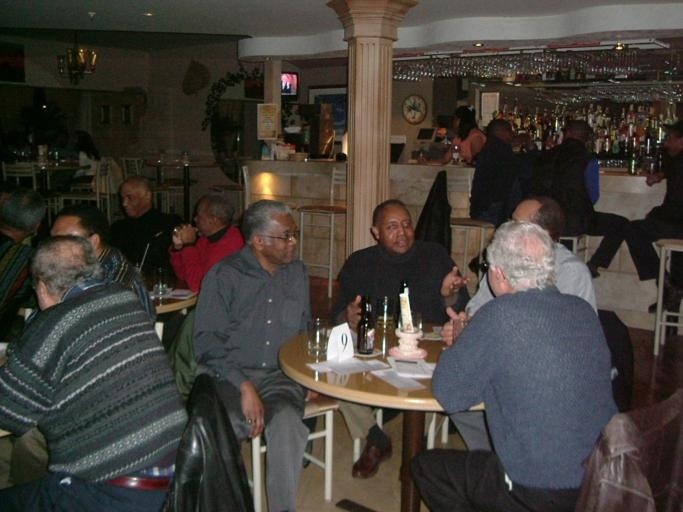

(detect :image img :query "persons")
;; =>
[469,117,538,273]
[331,200,469,477]
[531,119,629,279]
[440,194,597,348]
[409,221,619,510]
[417,106,488,166]
[69,130,100,177]
[0,175,244,343]
[619,121,682,336]
[0,234,188,512]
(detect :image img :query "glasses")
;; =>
[259,229,300,241]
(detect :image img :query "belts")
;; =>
[111,475,170,491]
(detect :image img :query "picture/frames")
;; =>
[305,83,347,146]
[401,94,428,125]
[255,102,280,141]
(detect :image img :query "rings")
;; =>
[248,419,251,423]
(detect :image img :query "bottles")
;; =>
[356,295,376,353]
[498,98,675,175]
[182,152,188,160]
[152,267,166,305]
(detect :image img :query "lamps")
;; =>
[57,46,97,84]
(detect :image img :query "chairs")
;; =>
[450,168,493,294]
[297,166,345,298]
[5,154,247,228]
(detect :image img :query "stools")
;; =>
[559,234,586,264]
[653,237,682,357]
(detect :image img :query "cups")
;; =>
[306,319,329,354]
[451,318,469,340]
[377,296,397,330]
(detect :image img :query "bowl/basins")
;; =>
[283,127,301,134]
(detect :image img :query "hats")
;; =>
[663,121,683,133]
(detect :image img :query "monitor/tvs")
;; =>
[279,72,298,96]
[415,127,436,142]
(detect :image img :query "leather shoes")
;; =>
[648,283,683,313]
[352,440,393,479]
